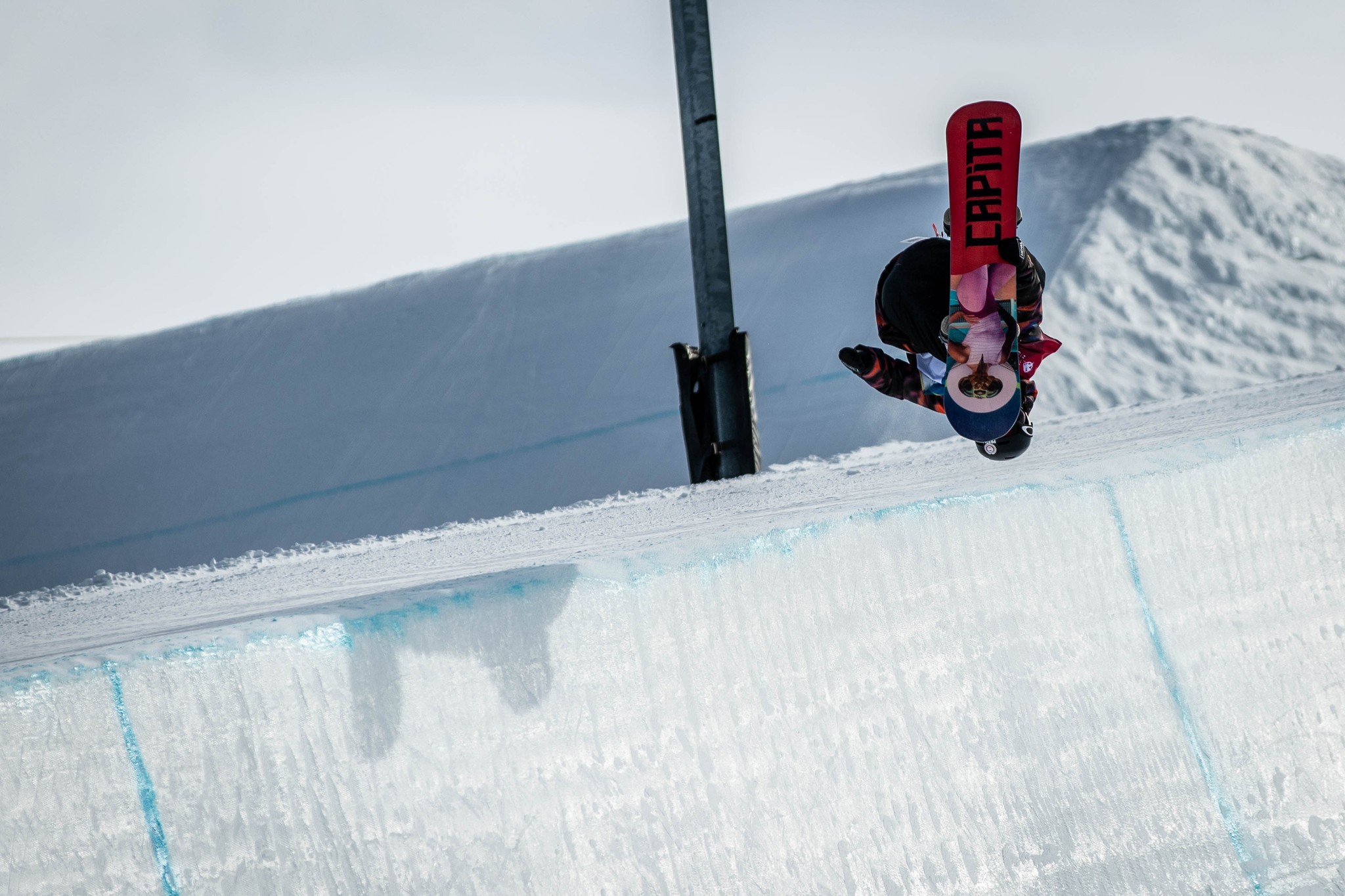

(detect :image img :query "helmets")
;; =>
[975,407,1035,461]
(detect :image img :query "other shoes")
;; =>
[1017,206,1023,227]
[944,209,951,225]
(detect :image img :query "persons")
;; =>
[838,205,1062,461]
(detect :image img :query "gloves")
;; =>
[998,237,1026,266]
[839,346,875,376]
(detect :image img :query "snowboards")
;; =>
[942,100,1022,443]
